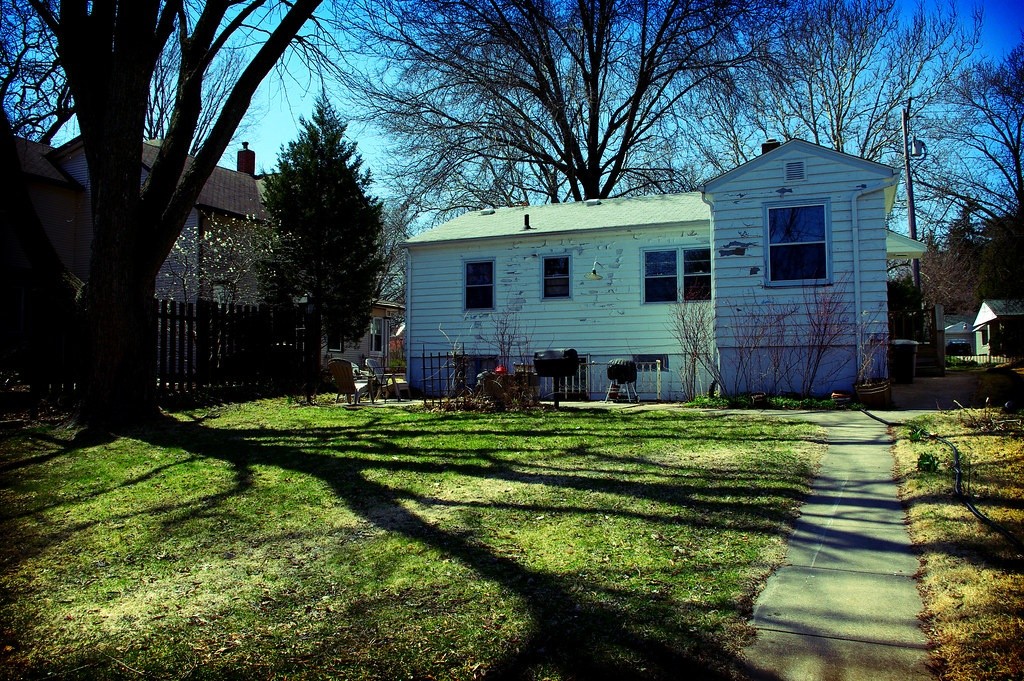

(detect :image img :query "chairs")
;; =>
[327,357,412,405]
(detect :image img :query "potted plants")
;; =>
[851,311,891,410]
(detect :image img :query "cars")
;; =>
[945,337,971,356]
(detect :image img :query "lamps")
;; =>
[584,261,606,280]
